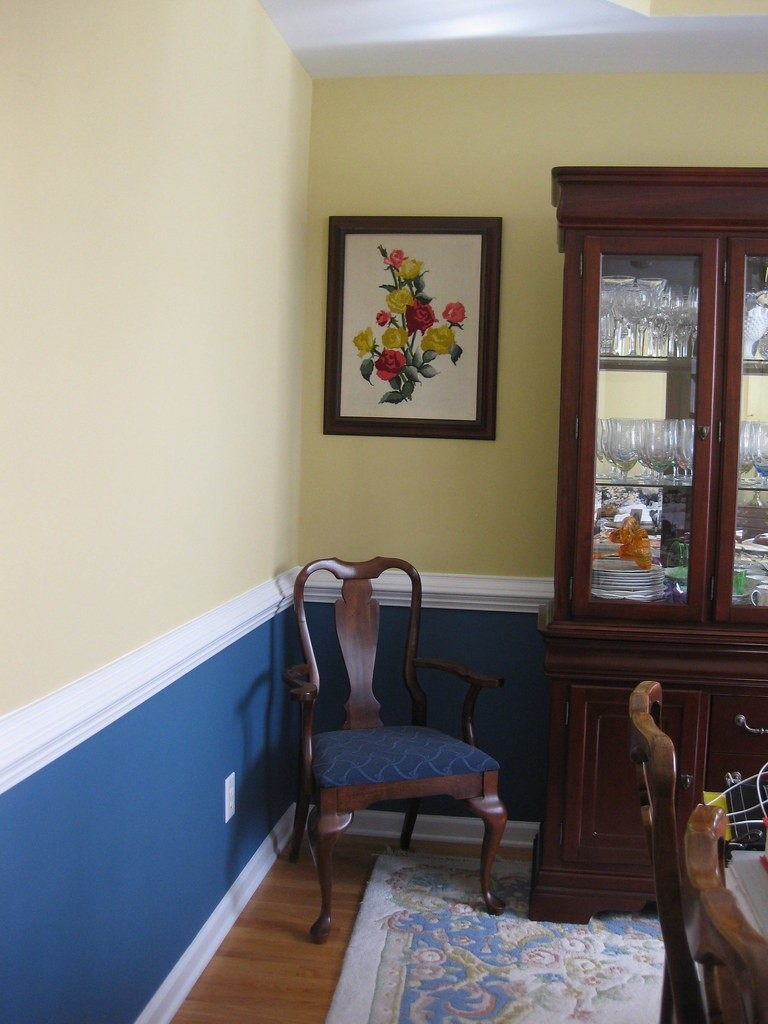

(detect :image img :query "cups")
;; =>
[732,562,768,606]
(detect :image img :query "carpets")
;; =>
[324,847,663,1024]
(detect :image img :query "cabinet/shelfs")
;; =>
[543,165,768,639]
[527,604,767,924]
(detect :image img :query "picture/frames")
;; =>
[323,215,504,442]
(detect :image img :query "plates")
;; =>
[591,554,668,602]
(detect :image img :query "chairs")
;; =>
[684,806,768,1024]
[627,679,707,1024]
[282,555,513,944]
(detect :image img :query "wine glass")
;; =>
[597,417,694,484]
[600,274,698,358]
[738,420,768,488]
